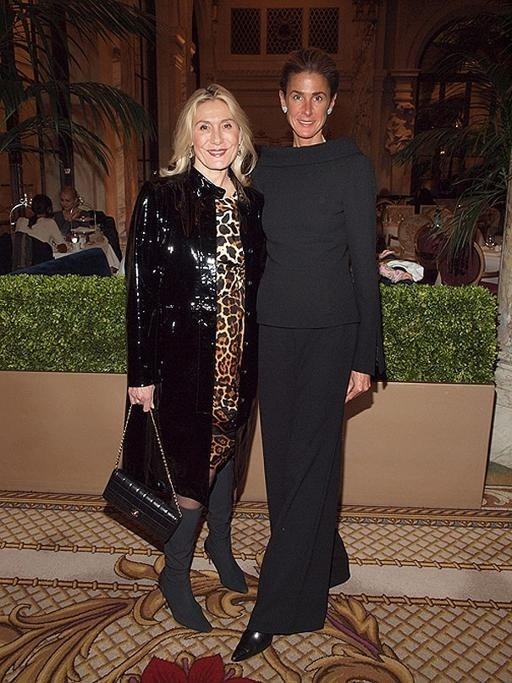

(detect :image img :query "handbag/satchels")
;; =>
[101,405,183,553]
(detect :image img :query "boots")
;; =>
[158,506,213,633]
[204,458,248,594]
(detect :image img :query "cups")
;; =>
[70,233,78,243]
[485,234,496,247]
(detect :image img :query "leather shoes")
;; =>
[231,629,273,662]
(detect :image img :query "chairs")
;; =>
[0,210,123,276]
[376,205,501,286]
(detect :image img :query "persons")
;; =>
[227,44,389,661]
[118,81,264,631]
[14,193,68,252]
[51,185,92,244]
